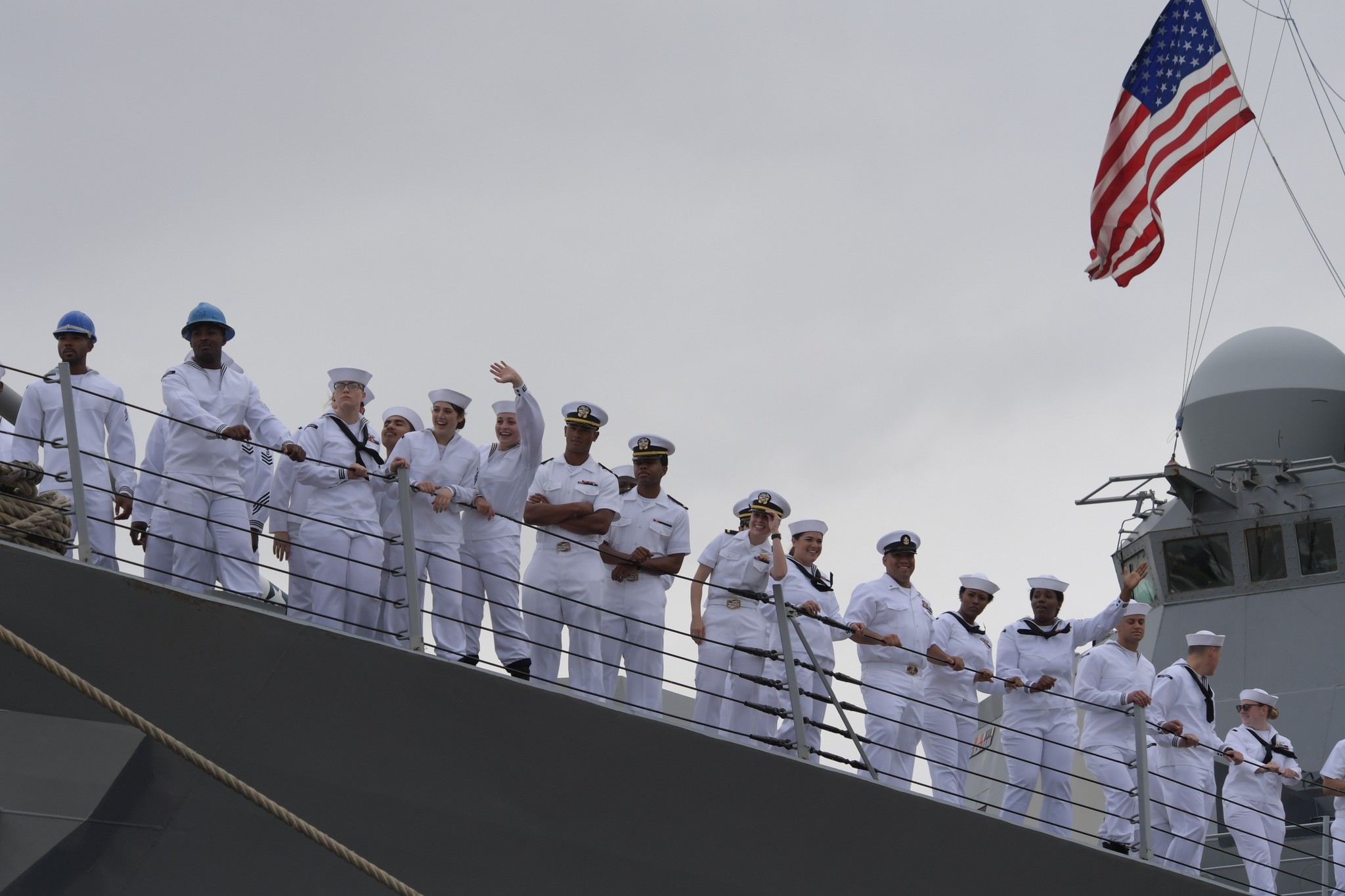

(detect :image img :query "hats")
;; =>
[959,572,1001,595]
[1122,599,1152,617]
[876,530,921,554]
[1185,630,1225,648]
[328,367,515,433]
[1027,573,1070,594]
[627,433,675,461]
[1239,687,1278,707]
[733,489,792,521]
[610,464,637,480]
[561,400,609,430]
[789,519,828,537]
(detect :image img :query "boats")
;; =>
[2,327,1345,896]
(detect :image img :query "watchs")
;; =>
[771,533,781,540]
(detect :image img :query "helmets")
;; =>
[181,301,236,342]
[52,311,98,344]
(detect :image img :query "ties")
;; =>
[947,611,986,635]
[1184,666,1215,724]
[1016,616,1071,639]
[787,557,835,592]
[1246,728,1298,766]
[328,416,385,482]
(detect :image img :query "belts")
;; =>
[707,597,758,612]
[861,660,924,678]
[534,541,598,552]
[603,569,661,581]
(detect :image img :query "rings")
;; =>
[962,664,964,666]
[540,501,542,503]
[693,634,694,635]
[439,499,442,501]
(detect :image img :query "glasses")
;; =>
[1236,704,1268,713]
[334,382,363,392]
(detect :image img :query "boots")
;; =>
[506,657,532,682]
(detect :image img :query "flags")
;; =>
[1084,0,1257,289]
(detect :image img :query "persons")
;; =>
[996,562,1150,837]
[522,401,622,703]
[920,573,1025,806]
[598,434,697,718]
[1221,689,1302,896]
[1320,738,1345,896]
[691,489,867,764]
[610,465,675,710]
[1073,598,1183,855]
[129,303,545,682]
[9,310,137,572]
[1149,631,1244,877]
[843,530,965,790]
